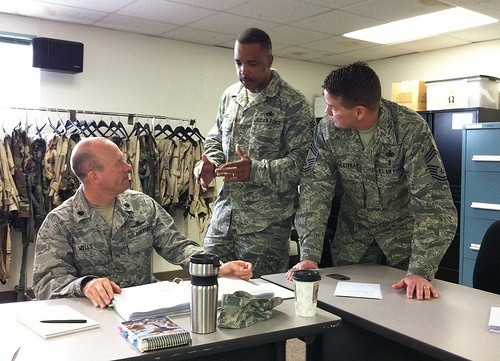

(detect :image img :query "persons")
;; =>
[285,61,459,301]
[32,136,253,309]
[194,28,317,280]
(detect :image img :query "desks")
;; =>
[0,274,343,361]
[262,262,500,361]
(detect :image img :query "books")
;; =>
[487,306,500,333]
[118,314,189,352]
[16,305,99,340]
[115,275,258,321]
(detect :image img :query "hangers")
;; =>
[0,107,206,148]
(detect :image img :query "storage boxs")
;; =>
[391,73,500,111]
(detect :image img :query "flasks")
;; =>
[188,254,221,335]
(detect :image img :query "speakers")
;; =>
[32,37,84,75]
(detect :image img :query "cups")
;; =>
[292,270,322,317]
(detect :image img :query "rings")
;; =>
[424,286,430,289]
[233,173,234,177]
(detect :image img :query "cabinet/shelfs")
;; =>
[297,108,500,291]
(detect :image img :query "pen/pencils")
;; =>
[39,319,87,324]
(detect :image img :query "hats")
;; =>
[218,291,283,329]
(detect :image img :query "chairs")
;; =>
[473,220,500,296]
